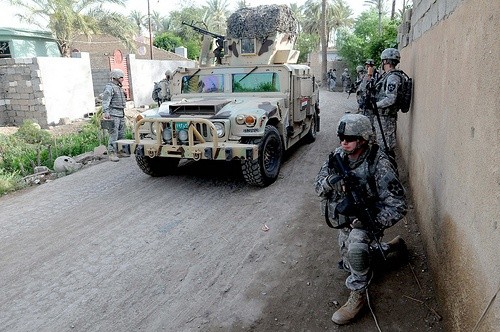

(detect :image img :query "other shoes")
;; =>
[118,152,126,158]
[331,290,372,325]
[108,153,119,162]
[389,235,410,265]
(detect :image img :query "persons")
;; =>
[325,64,369,94]
[313,112,411,325]
[360,48,404,161]
[156,69,172,106]
[102,70,130,162]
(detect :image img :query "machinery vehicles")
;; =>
[111,24,320,187]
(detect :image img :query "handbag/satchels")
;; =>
[152,82,158,102]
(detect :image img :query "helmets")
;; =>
[366,59,374,64]
[336,114,372,141]
[344,68,348,71]
[112,69,124,79]
[381,48,400,63]
[165,70,171,75]
[356,66,364,71]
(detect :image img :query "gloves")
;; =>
[328,174,345,191]
[351,218,362,229]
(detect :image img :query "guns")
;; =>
[181,19,225,47]
[330,153,388,261]
[362,68,377,116]
[347,79,361,97]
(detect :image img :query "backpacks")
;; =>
[395,70,413,113]
